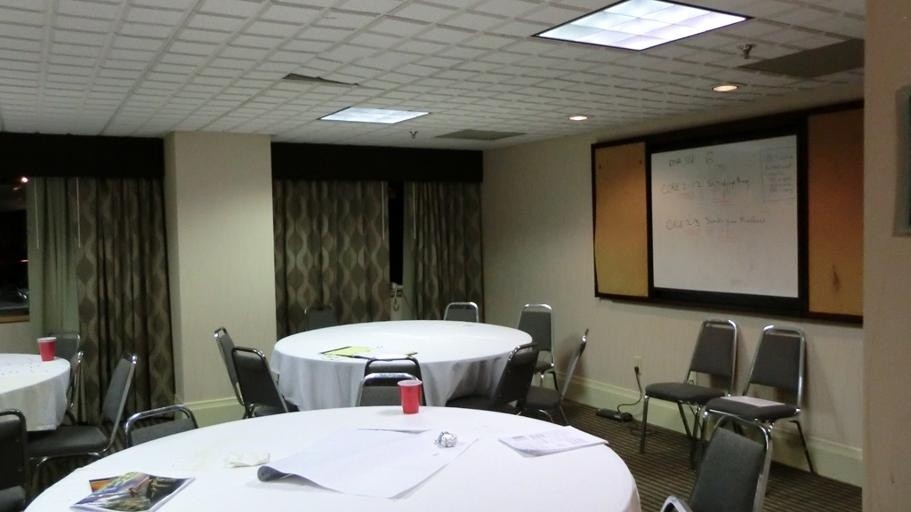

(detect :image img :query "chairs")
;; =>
[355,373,423,406]
[28,351,138,495]
[640,319,738,469]
[214,327,259,419]
[364,358,426,406]
[231,346,300,418]
[67,351,84,410]
[516,329,589,425]
[660,494,693,512]
[705,324,815,475]
[123,404,199,449]
[443,301,476,322]
[0,408,27,512]
[304,304,337,331]
[516,303,559,391]
[48,331,80,425]
[687,414,774,512]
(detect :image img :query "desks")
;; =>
[0,353,71,432]
[23,406,642,512]
[269,319,533,411]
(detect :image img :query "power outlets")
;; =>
[631,357,642,375]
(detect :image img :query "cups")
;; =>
[396,379,422,415]
[36,336,57,362]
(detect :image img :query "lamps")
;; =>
[10,176,29,192]
[15,259,28,302]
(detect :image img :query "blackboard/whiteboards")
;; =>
[646,115,808,315]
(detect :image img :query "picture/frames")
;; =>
[893,85,911,236]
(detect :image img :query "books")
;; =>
[70,469,195,512]
